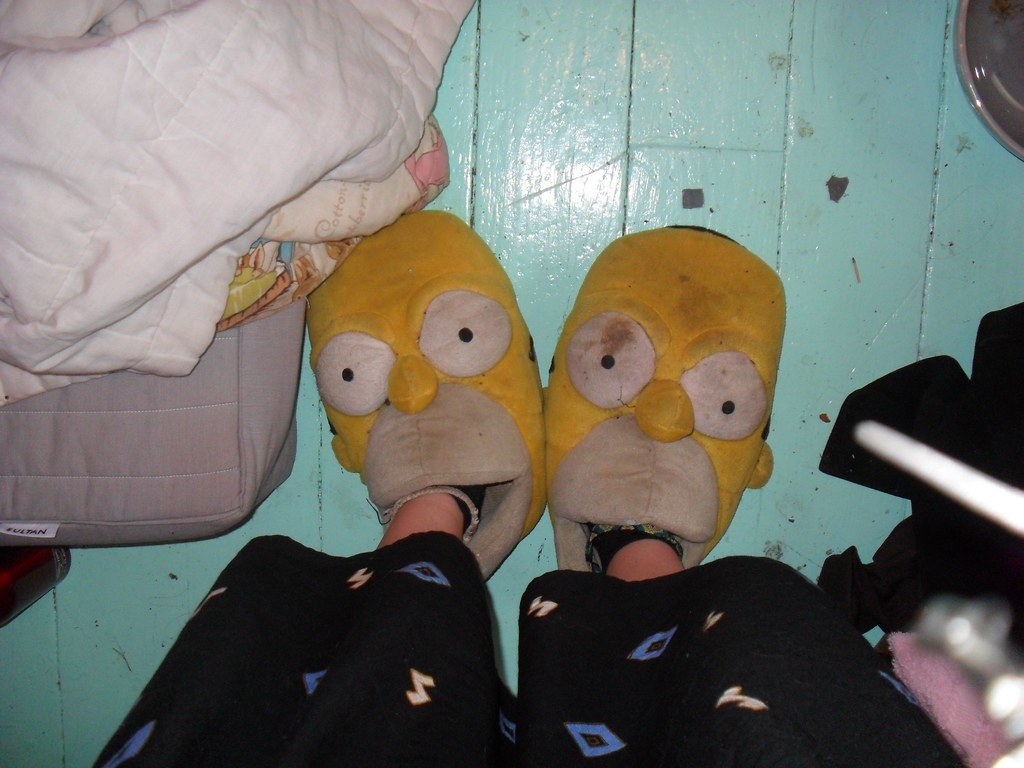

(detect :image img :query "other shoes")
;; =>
[546,225,786,571]
[307,210,544,582]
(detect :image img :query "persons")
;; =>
[92,212,1024,768]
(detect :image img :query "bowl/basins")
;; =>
[957,0,1024,163]
